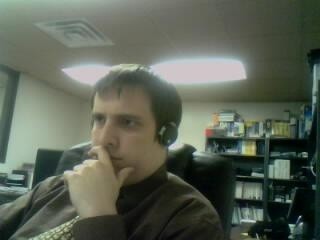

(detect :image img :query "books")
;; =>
[218,109,289,225]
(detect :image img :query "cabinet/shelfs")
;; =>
[206,137,317,226]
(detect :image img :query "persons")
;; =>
[0,64,223,240]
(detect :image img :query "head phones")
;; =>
[157,121,178,146]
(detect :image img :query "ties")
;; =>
[27,215,80,240]
